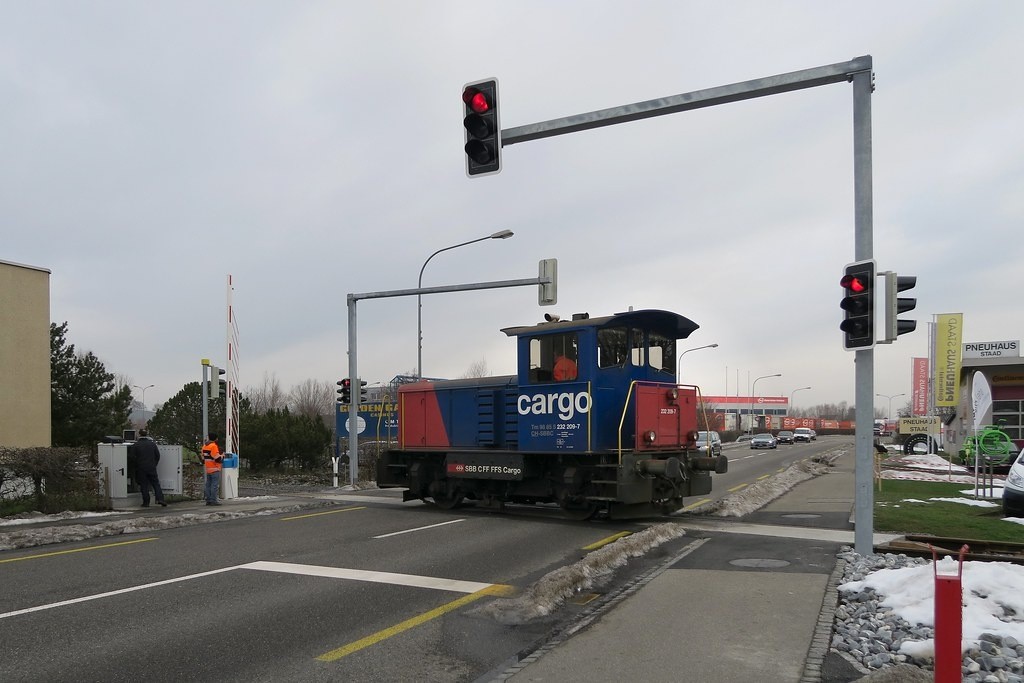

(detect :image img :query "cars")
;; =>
[750,434,777,449]
[776,431,794,445]
[693,431,723,455]
[811,430,817,439]
[968,442,1024,518]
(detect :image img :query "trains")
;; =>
[375,306,728,521]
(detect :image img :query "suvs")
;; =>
[696,431,722,457]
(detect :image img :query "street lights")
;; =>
[790,387,811,431]
[678,343,718,388]
[418,230,514,380]
[876,393,905,437]
[752,374,781,440]
[134,385,155,425]
[679,344,719,387]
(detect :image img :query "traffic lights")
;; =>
[461,77,503,178]
[892,273,917,340]
[337,378,350,404]
[840,259,877,350]
[361,380,367,403]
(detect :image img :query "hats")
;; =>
[208,432,217,441]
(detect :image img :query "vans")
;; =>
[794,428,811,443]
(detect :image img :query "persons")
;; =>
[201,432,225,506]
[552,345,577,381]
[128,429,167,507]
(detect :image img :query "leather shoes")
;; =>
[206,500,221,506]
[141,503,148,507]
[158,500,168,506]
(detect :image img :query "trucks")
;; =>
[874,420,897,436]
